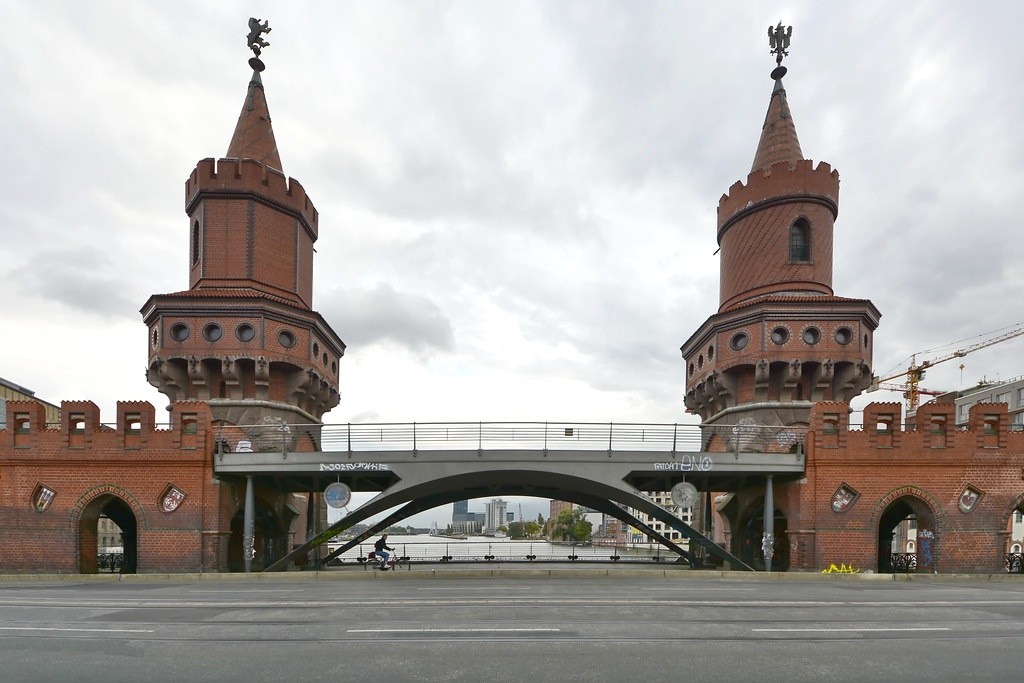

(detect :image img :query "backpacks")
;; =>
[375,541,380,547]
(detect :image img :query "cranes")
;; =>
[867,323,1024,418]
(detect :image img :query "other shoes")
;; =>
[384,564,391,568]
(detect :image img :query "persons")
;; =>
[376,534,395,569]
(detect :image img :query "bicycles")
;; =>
[365,548,410,570]
